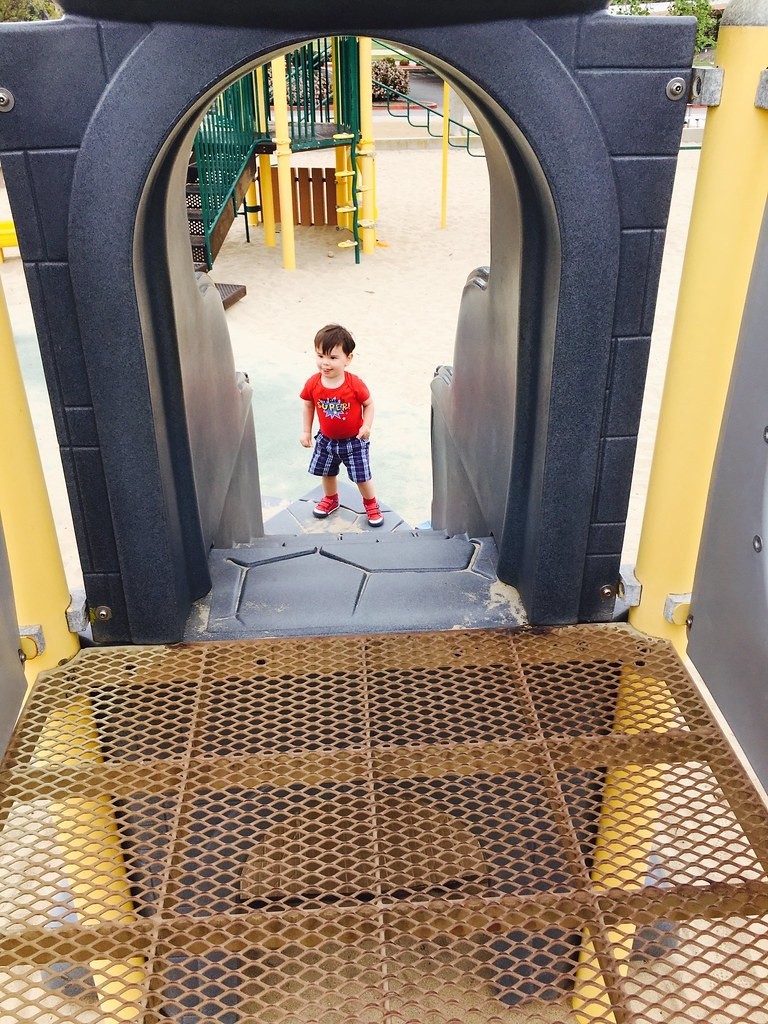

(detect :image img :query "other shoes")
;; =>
[312,497,340,518]
[364,503,384,527]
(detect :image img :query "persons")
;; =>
[300,323,384,528]
